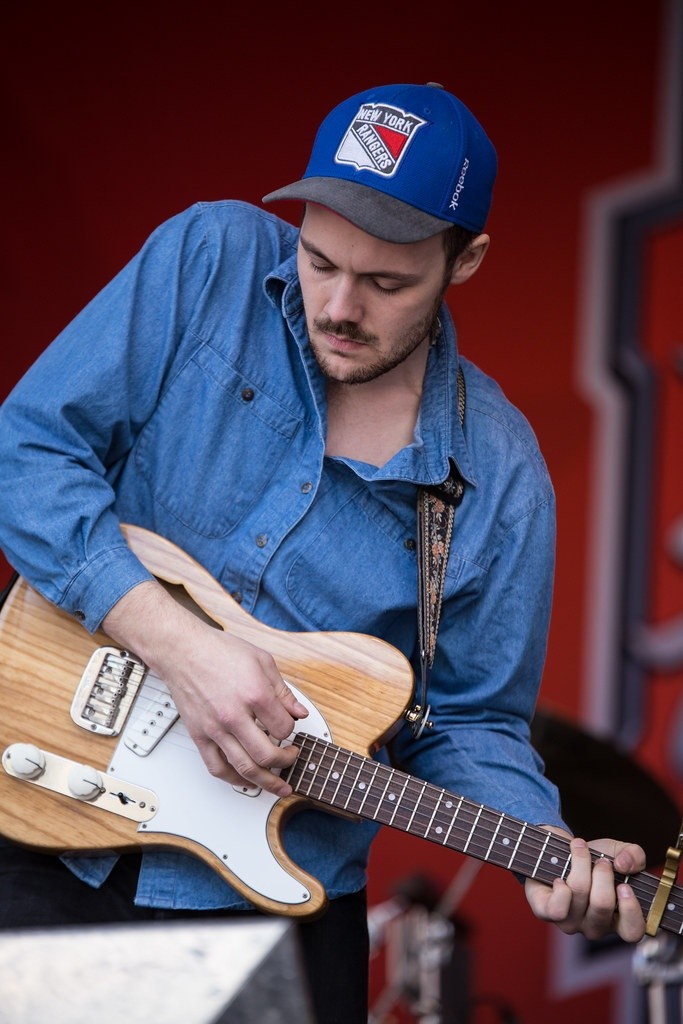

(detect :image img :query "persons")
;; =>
[0,82,646,1024]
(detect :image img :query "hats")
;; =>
[262,80,496,245]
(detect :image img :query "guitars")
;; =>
[0,518,682,973]
